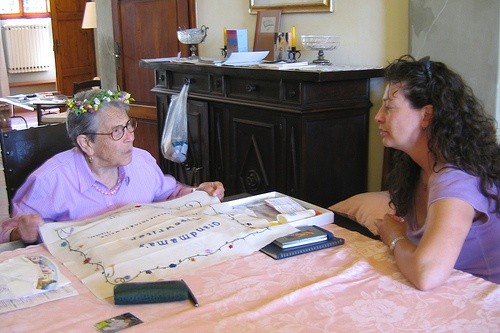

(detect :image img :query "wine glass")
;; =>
[177,26,208,61]
[301,34,337,65]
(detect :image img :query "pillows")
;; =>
[328,191,399,236]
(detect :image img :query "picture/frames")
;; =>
[249,0,333,15]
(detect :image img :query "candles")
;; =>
[292,26,296,47]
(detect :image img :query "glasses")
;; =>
[418,56,432,80]
[79,117,138,141]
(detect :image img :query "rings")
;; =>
[400,216,408,223]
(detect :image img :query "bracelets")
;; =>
[191,187,197,193]
[389,236,409,256]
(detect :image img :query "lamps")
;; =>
[81,2,97,29]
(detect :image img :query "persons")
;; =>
[373,54,500,290]
[0,90,225,244]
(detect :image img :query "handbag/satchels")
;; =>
[161,82,190,163]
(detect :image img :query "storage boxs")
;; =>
[222,191,334,226]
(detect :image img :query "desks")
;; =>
[0,92,71,128]
[0,224,500,333]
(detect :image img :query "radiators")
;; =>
[2,26,50,74]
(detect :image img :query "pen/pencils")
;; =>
[273,32,291,44]
[181,279,199,307]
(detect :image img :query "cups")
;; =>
[0,101,14,131]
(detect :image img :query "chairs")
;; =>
[0,103,29,129]
[33,103,70,127]
[0,123,76,215]
[73,80,101,99]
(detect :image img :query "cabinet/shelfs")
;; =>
[139,61,385,209]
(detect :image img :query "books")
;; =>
[259,226,344,260]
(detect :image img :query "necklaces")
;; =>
[92,184,120,195]
[423,179,427,209]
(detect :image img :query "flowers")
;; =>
[64,85,134,116]
